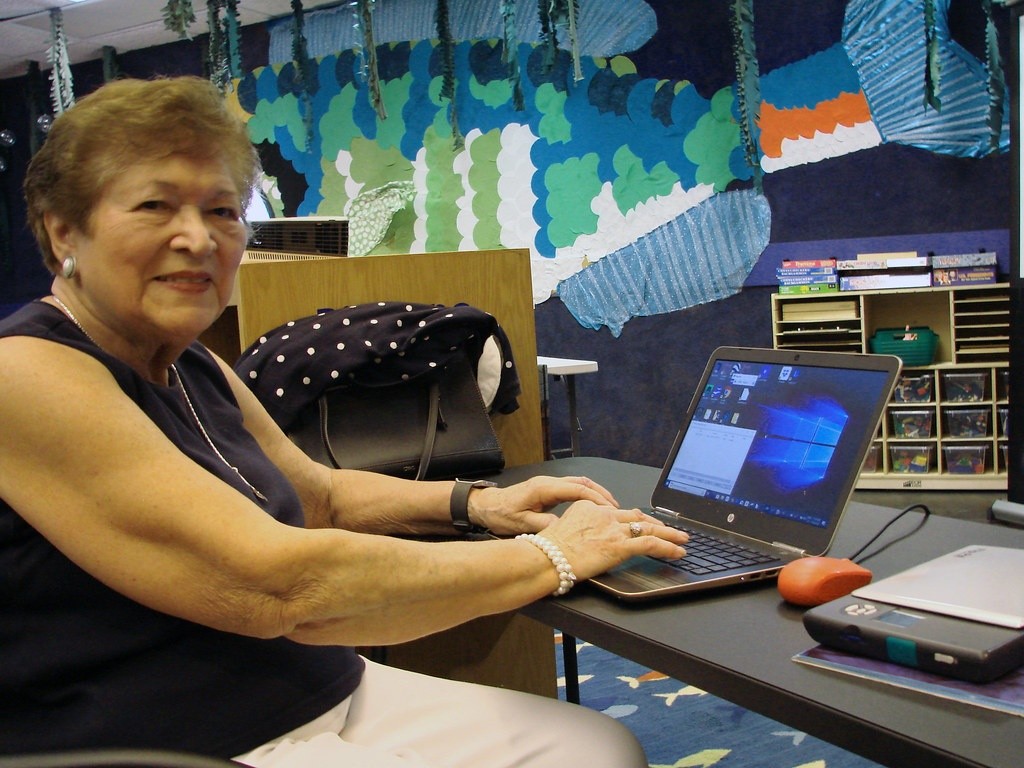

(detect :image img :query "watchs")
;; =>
[450,478,498,534]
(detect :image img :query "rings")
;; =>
[630,522,641,537]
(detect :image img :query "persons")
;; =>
[0,76,688,765]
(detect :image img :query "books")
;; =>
[782,301,858,320]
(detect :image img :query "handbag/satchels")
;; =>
[285,357,506,482]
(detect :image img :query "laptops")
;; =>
[486,346,904,605]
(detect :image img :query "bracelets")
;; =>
[515,533,576,596]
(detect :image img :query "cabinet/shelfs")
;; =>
[770,282,1011,489]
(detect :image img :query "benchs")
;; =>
[538,356,598,457]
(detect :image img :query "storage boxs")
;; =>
[945,410,990,438]
[889,446,934,474]
[837,258,932,291]
[894,374,933,404]
[889,409,934,440]
[932,251,998,286]
[942,444,988,474]
[941,372,987,401]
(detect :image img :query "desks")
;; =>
[407,455,1024,768]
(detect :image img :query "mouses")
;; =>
[777,556,873,607]
